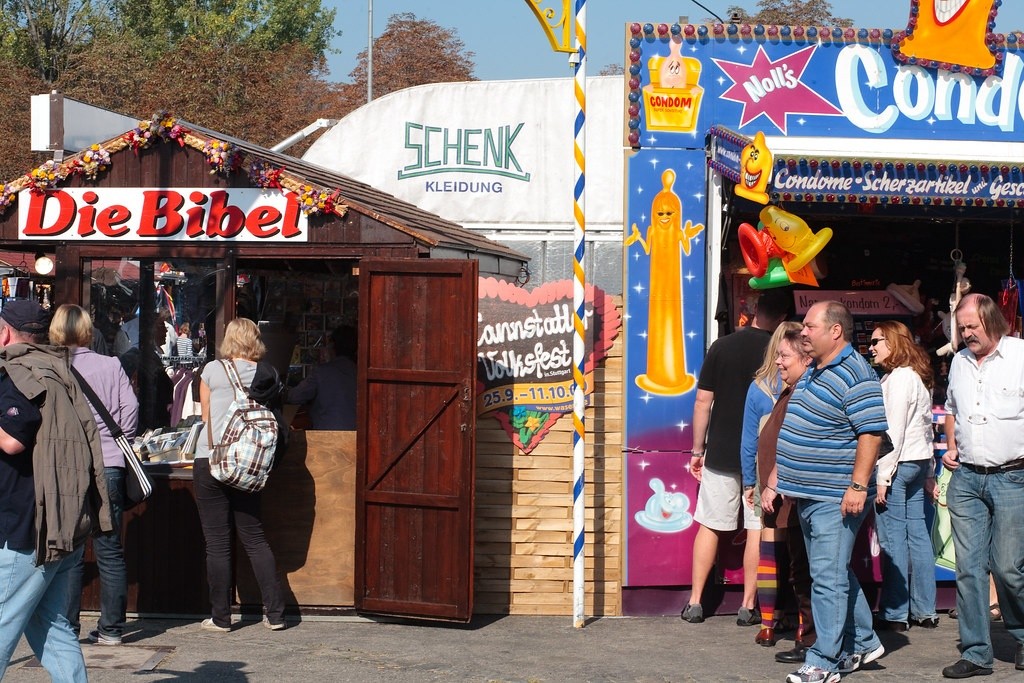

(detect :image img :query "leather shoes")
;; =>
[755,628,776,646]
[775,644,809,663]
[773,623,799,641]
[797,629,804,640]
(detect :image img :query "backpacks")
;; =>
[207,358,280,494]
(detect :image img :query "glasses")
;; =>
[871,338,888,346]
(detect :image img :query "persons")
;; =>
[48,305,140,645]
[943,293,1024,678]
[869,321,939,631]
[176,321,193,357]
[101,321,130,356]
[122,313,137,323]
[157,308,178,366]
[193,317,288,631]
[681,289,796,626]
[741,322,813,647]
[288,325,358,429]
[776,300,889,683]
[92,326,109,356]
[757,332,821,662]
[0,300,89,683]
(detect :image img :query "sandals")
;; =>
[948,603,1001,620]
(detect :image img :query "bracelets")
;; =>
[765,485,776,493]
[691,449,704,456]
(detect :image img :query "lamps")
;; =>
[34,250,54,276]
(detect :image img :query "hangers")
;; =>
[159,356,205,371]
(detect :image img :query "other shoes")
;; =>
[202,618,232,631]
[873,617,940,631]
[265,618,285,630]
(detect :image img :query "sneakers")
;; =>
[942,660,993,679]
[88,628,122,645]
[787,664,841,683]
[832,644,885,672]
[736,608,761,626]
[681,601,705,623]
[1014,643,1024,670]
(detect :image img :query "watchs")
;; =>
[850,480,870,492]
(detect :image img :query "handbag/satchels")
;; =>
[115,436,157,510]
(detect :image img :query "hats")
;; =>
[0,300,51,333]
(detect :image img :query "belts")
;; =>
[960,459,1024,475]
